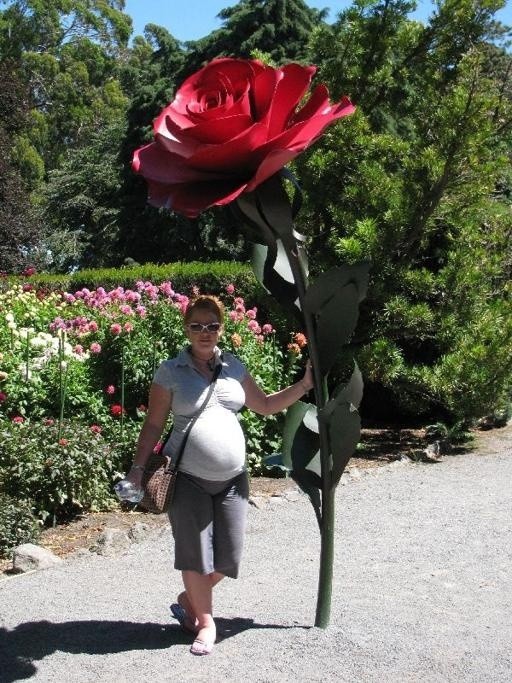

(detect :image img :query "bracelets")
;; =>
[130,465,146,473]
[298,378,311,399]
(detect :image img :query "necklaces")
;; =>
[191,351,217,373]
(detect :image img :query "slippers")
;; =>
[170,604,195,634]
[190,639,217,655]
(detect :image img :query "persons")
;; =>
[121,294,316,655]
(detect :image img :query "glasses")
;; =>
[188,322,221,333]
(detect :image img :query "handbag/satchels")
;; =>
[136,454,178,515]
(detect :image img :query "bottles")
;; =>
[113,479,145,503]
